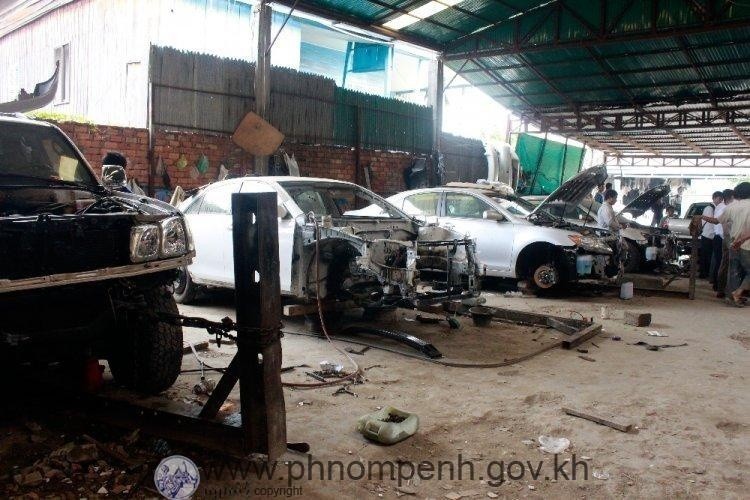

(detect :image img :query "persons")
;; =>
[103,152,131,192]
[594,182,750,308]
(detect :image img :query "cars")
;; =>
[0,115,193,394]
[341,165,630,297]
[519,184,678,273]
[662,202,716,256]
[164,175,483,313]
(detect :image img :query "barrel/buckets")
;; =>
[620,281,635,301]
[576,255,593,275]
[645,246,657,262]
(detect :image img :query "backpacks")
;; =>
[695,204,715,228]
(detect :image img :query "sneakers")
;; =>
[700,274,744,307]
